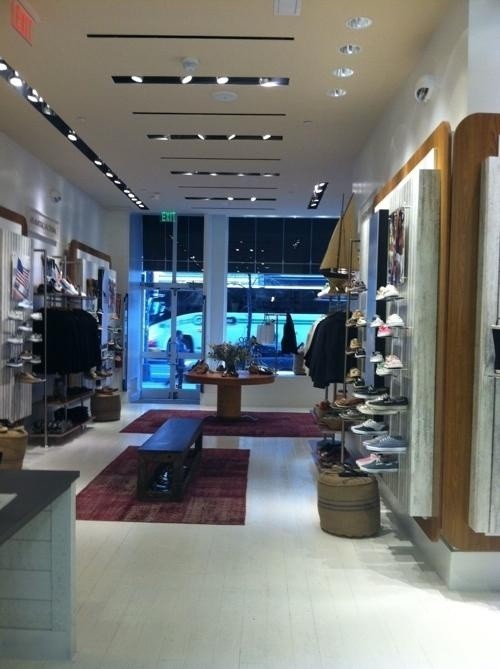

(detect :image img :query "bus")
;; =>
[143,284,348,362]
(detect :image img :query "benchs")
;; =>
[136,416,204,503]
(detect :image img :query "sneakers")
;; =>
[317,443,348,466]
[216,365,239,377]
[15,371,47,384]
[188,359,209,374]
[5,310,42,369]
[248,364,273,376]
[335,280,409,477]
[85,367,113,379]
[85,307,96,313]
[96,308,104,314]
[108,325,124,334]
[97,325,104,331]
[100,339,124,362]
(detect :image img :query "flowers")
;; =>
[208,337,260,362]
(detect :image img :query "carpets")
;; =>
[119,408,336,438]
[75,445,251,526]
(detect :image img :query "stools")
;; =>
[90,391,121,422]
[316,464,382,537]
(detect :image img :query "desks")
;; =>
[183,370,275,424]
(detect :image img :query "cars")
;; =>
[239,342,296,373]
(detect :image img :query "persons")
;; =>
[166,330,191,389]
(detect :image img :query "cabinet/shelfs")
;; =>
[32,291,96,438]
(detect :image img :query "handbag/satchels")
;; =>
[256,324,275,346]
